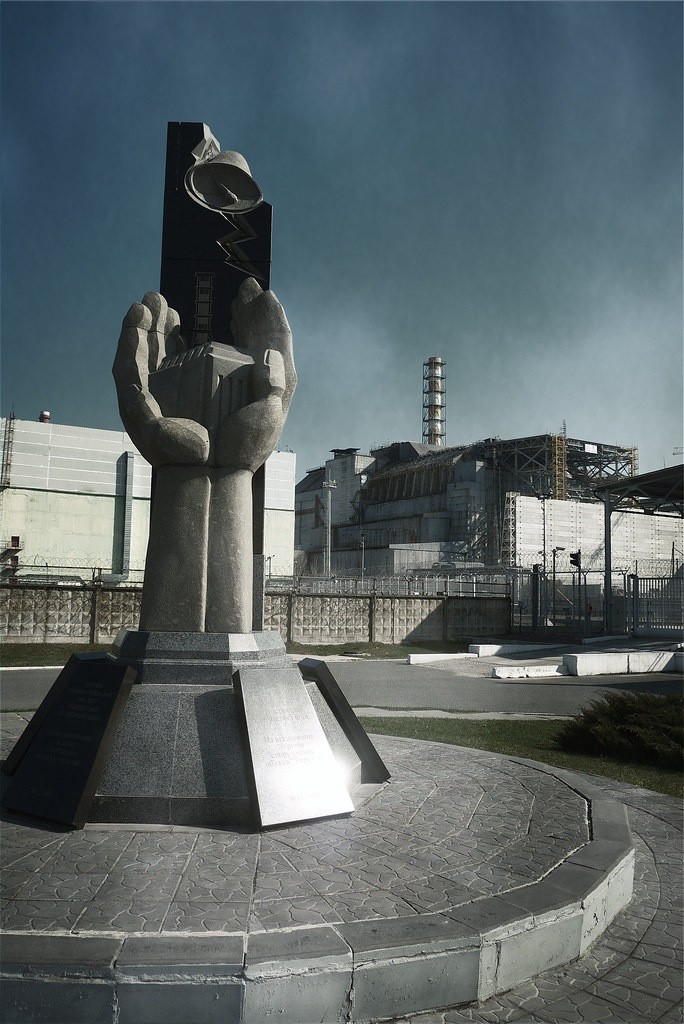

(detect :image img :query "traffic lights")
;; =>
[568,549,581,567]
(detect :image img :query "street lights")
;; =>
[536,488,554,626]
[359,534,367,590]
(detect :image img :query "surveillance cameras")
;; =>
[556,547,565,551]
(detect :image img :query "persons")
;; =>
[112,276,298,634]
